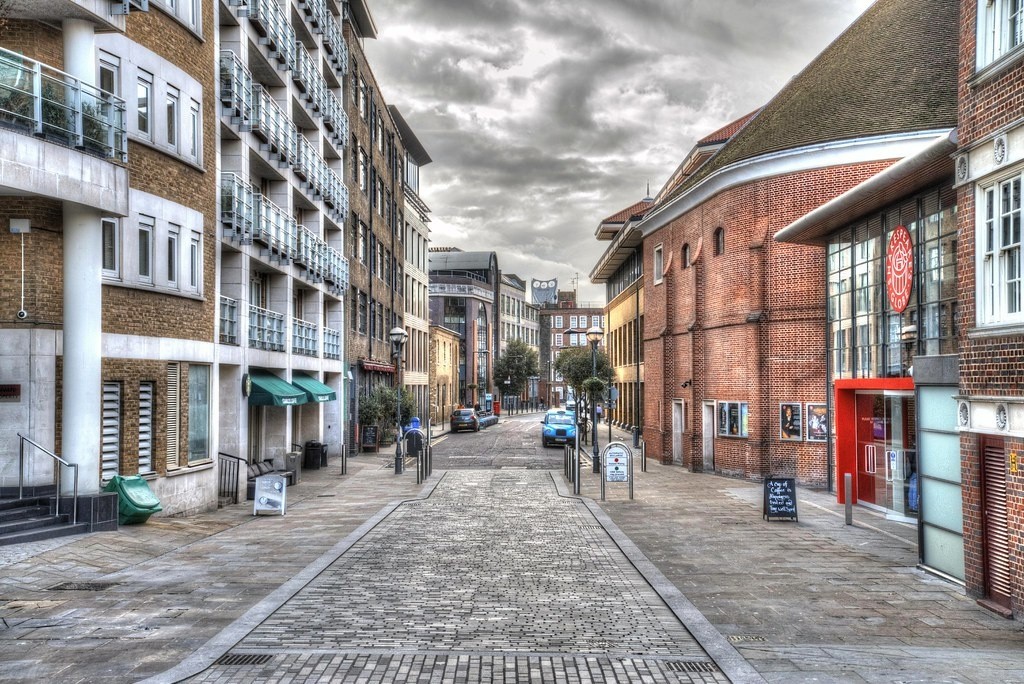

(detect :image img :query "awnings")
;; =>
[292,371,337,402]
[249,369,307,406]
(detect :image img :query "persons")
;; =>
[474,402,482,411]
[597,404,602,424]
[466,402,473,408]
[457,402,465,409]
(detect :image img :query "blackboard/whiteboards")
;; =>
[763,477,798,517]
[360,425,379,447]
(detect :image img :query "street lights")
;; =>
[472,350,490,408]
[507,355,524,417]
[562,329,595,446]
[586,326,604,474]
[389,327,409,475]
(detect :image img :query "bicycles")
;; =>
[580,419,593,433]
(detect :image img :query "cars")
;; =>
[540,408,576,448]
[566,400,577,410]
[450,408,480,433]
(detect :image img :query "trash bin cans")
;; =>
[305,441,322,470]
[402,416,420,432]
[103,473,162,525]
